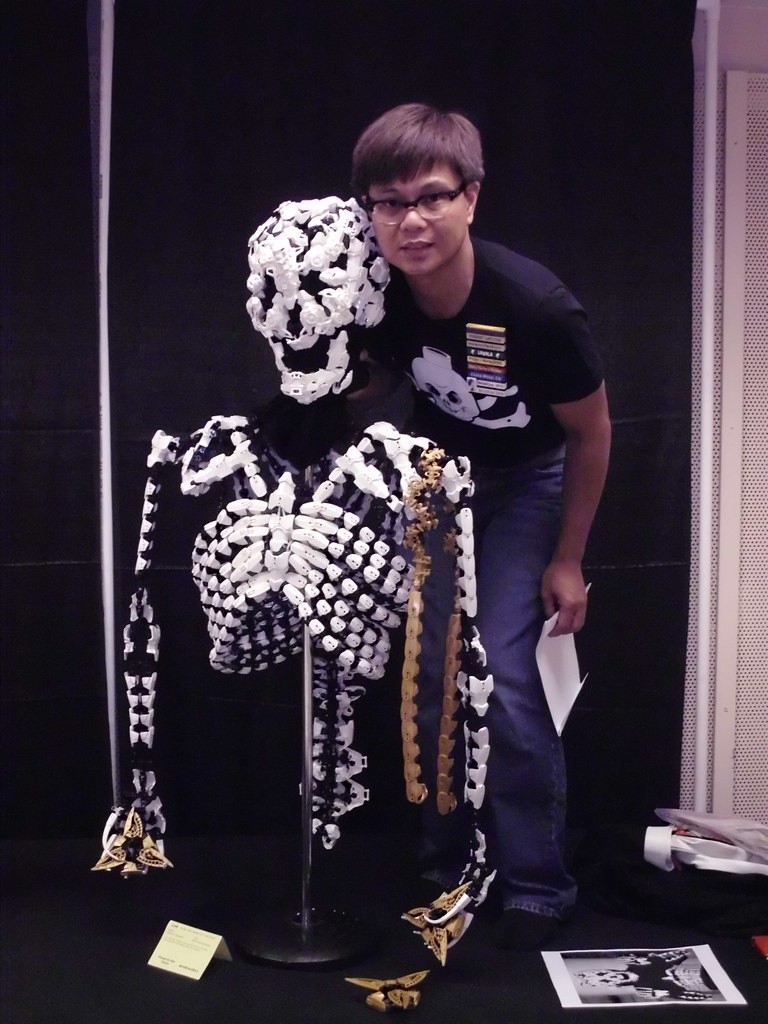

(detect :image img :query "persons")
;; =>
[344,105,613,954]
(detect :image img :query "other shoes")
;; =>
[501,909,548,951]
[406,876,444,905]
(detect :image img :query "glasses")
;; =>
[362,181,467,225]
